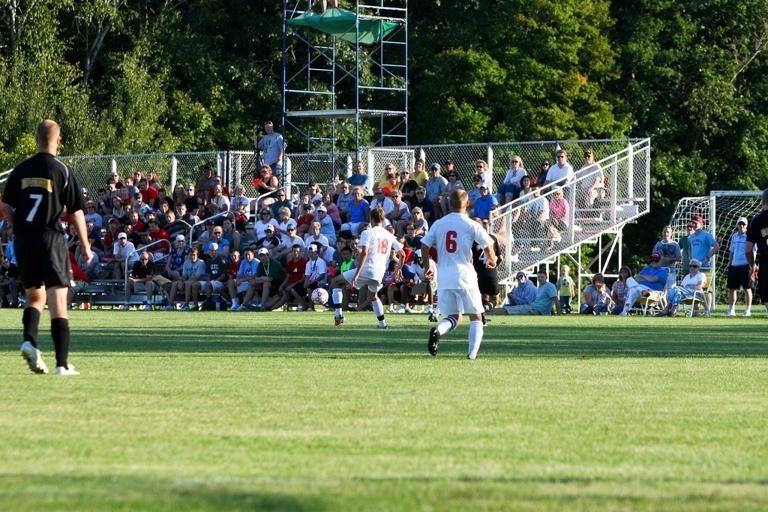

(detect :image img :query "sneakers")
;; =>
[691,309,752,318]
[386,305,439,321]
[143,304,199,311]
[20,341,48,374]
[55,363,80,376]
[620,301,676,316]
[123,306,129,311]
[427,327,440,356]
[225,302,305,312]
[377,325,387,329]
[334,316,344,326]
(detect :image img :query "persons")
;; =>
[257,118,285,183]
[746,188,768,306]
[331,207,405,328]
[420,188,497,361]
[0,117,94,376]
[490,216,755,316]
[2,150,603,314]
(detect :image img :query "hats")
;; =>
[479,184,488,189]
[414,249,423,258]
[594,273,604,281]
[257,247,268,255]
[691,215,703,223]
[737,217,749,225]
[118,232,127,239]
[303,180,327,212]
[431,160,453,170]
[176,235,184,240]
[650,252,660,259]
[516,272,524,278]
[208,243,218,250]
[213,226,222,236]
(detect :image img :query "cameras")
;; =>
[256,126,263,131]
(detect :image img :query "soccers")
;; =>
[312,288,329,306]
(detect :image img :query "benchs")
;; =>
[503,197,645,262]
[80,198,264,305]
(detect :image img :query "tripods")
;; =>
[240,132,264,196]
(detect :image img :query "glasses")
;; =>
[261,193,296,232]
[471,166,482,182]
[448,174,455,178]
[541,155,590,166]
[374,168,425,214]
[511,160,518,164]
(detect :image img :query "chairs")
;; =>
[624,267,670,316]
[680,267,714,318]
